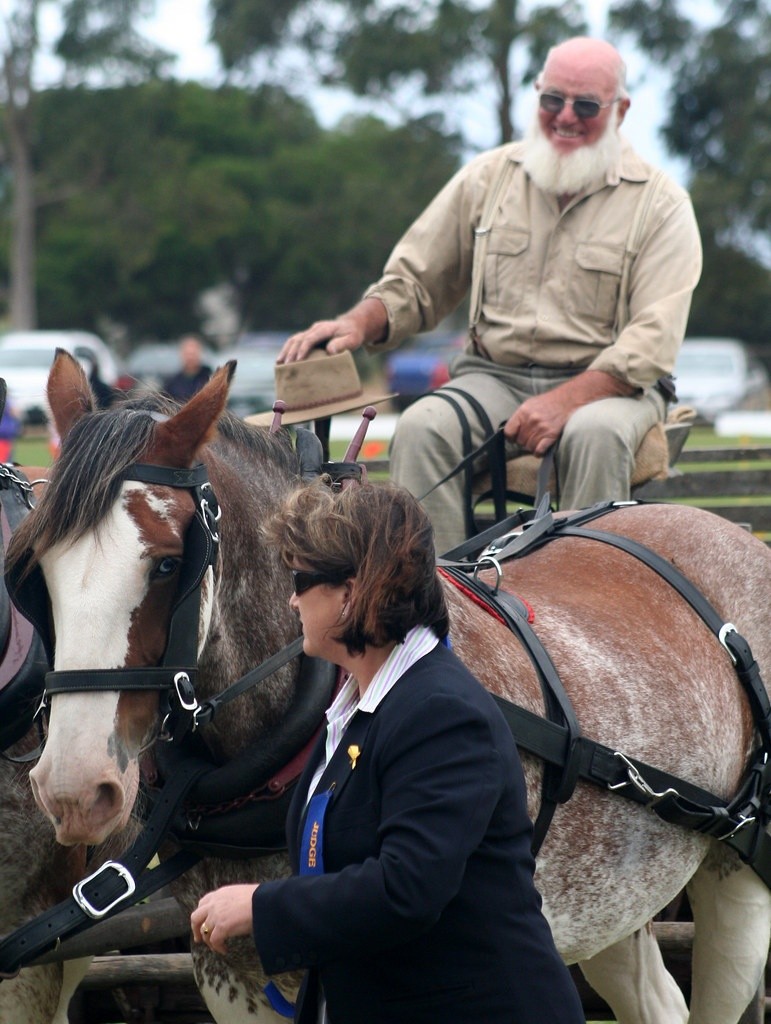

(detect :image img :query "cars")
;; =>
[0,327,121,425]
[112,332,295,422]
[672,339,768,425]
[384,327,472,410]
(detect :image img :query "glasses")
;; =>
[292,566,356,596]
[539,94,620,119]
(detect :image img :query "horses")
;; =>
[0,343,770,1023]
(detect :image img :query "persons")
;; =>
[275,35,703,565]
[160,333,216,408]
[189,474,586,1024]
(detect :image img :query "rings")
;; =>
[201,923,211,937]
